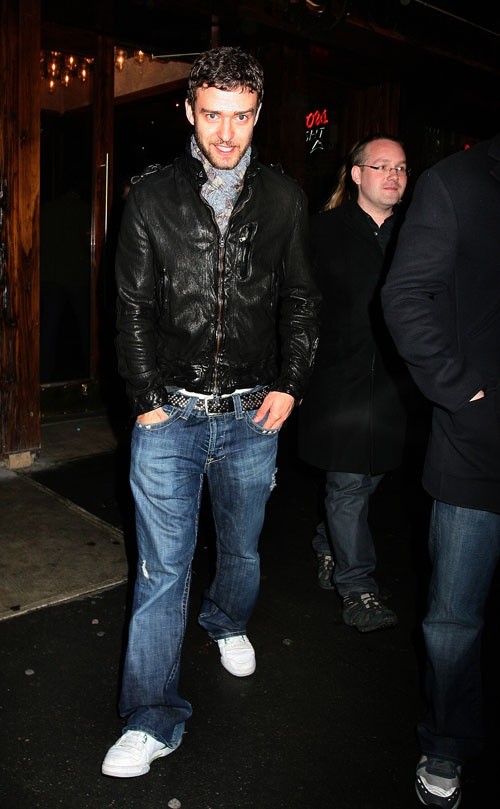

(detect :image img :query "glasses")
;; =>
[357,163,406,177]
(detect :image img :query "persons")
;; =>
[381,141,500,809]
[299,132,409,634]
[98,46,320,778]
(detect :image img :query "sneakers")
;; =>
[102,729,184,778]
[342,593,398,632]
[414,753,461,809]
[218,634,257,677]
[318,551,335,589]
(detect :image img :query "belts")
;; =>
[160,384,273,416]
